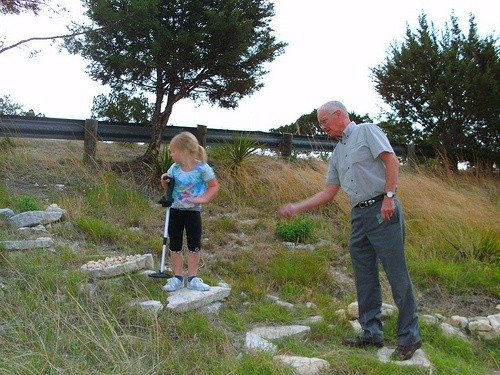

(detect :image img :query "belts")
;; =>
[354,194,384,208]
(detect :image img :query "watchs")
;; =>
[384,191,396,198]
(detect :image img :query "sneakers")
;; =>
[187,277,210,291]
[163,277,184,291]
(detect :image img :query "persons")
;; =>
[161,132,219,291]
[278,100,423,361]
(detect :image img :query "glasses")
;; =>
[319,112,335,128]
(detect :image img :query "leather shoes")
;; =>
[389,337,423,361]
[342,335,384,349]
